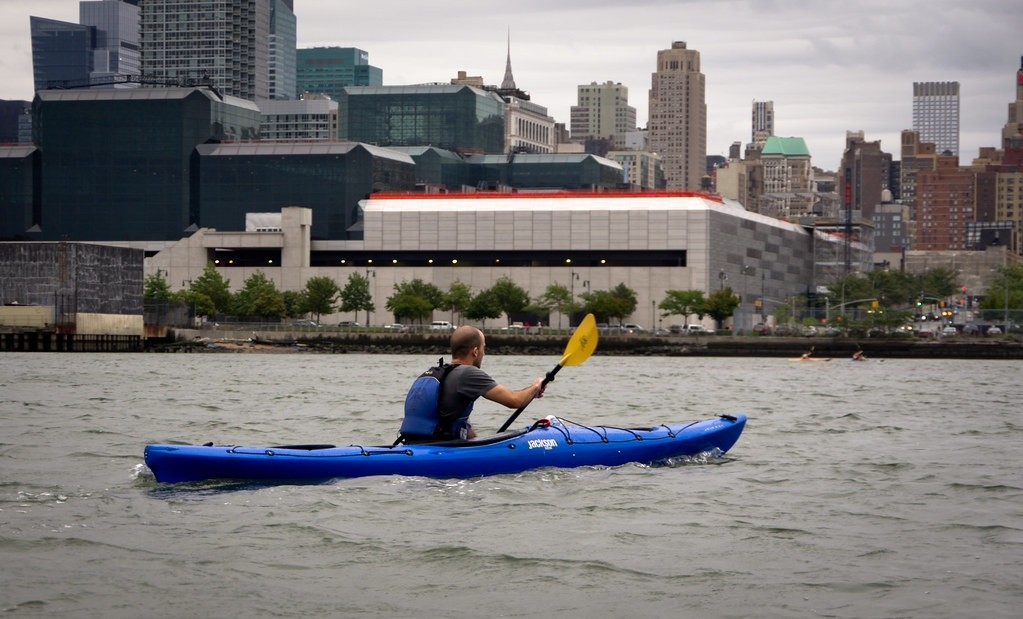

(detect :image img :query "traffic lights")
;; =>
[917,300,922,307]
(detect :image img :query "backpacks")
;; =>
[400,363,470,443]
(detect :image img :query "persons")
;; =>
[398,325,547,446]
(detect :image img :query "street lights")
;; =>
[489,258,501,291]
[451,258,458,325]
[599,258,611,295]
[428,257,437,290]
[364,258,375,330]
[180,280,200,327]
[990,268,1009,336]
[566,256,582,330]
[841,270,861,315]
[158,266,169,287]
[391,257,398,295]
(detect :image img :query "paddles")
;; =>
[496,311,599,435]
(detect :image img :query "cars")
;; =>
[753,322,771,336]
[384,324,402,330]
[773,324,912,337]
[596,321,705,337]
[914,325,932,338]
[986,327,1004,338]
[942,326,958,337]
[338,320,361,329]
[290,320,323,328]
[963,324,980,336]
[427,320,452,333]
[502,325,524,333]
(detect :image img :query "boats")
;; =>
[142,413,752,484]
